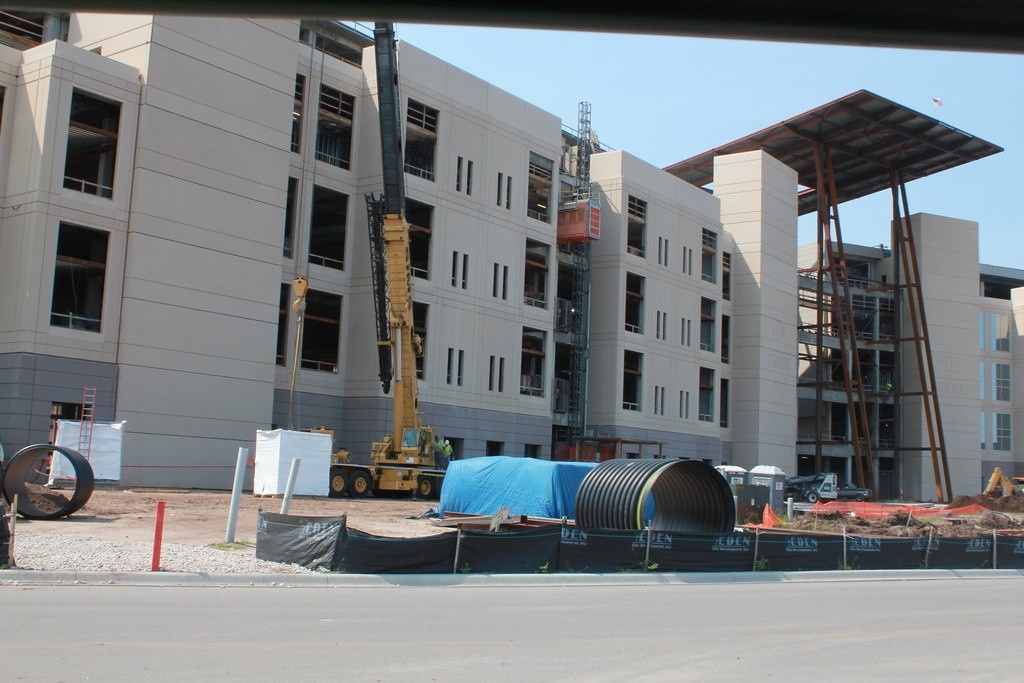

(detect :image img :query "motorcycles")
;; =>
[806,472,873,503]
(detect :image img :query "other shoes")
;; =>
[433,466,438,470]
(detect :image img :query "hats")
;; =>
[435,435,440,439]
[445,440,450,444]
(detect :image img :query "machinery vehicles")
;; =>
[983,466,1024,497]
[287,20,446,501]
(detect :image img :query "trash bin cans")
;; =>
[749,464,786,510]
[714,465,749,486]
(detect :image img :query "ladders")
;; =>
[78,383,97,461]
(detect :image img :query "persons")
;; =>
[434,435,454,469]
[887,381,893,392]
